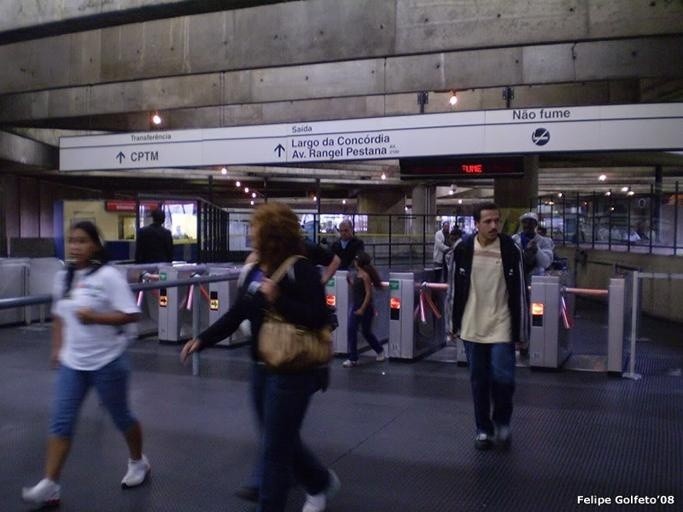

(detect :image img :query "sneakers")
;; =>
[376,348,385,362]
[474,425,512,449]
[121,452,151,487]
[22,479,61,506]
[303,470,341,512]
[342,359,359,368]
[233,486,259,501]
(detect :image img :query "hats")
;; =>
[519,212,538,221]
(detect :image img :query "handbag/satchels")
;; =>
[258,320,333,368]
[523,248,535,274]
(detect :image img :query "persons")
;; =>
[135,209,172,264]
[179,203,555,512]
[21,221,151,507]
